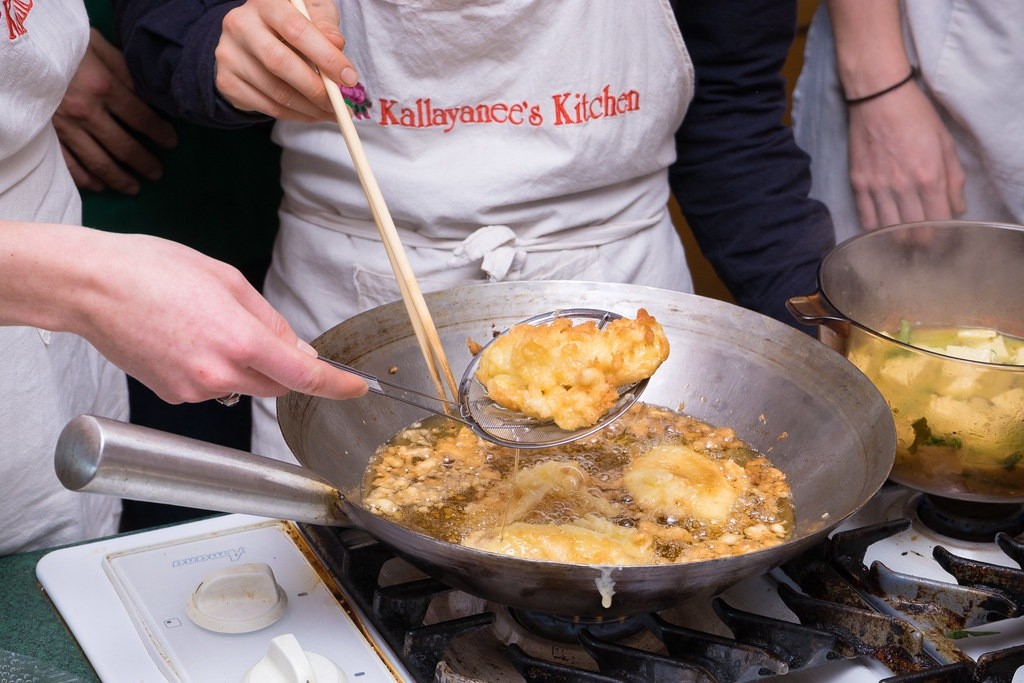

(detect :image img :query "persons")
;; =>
[122,0,836,468]
[1,1,367,553]
[793,0,1024,231]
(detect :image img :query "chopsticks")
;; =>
[292,0,462,431]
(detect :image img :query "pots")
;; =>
[55,280,899,620]
[784,223,1023,502]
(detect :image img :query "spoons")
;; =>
[317,308,650,449]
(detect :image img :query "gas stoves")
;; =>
[33,482,1023,683]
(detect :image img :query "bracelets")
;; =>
[839,65,916,106]
